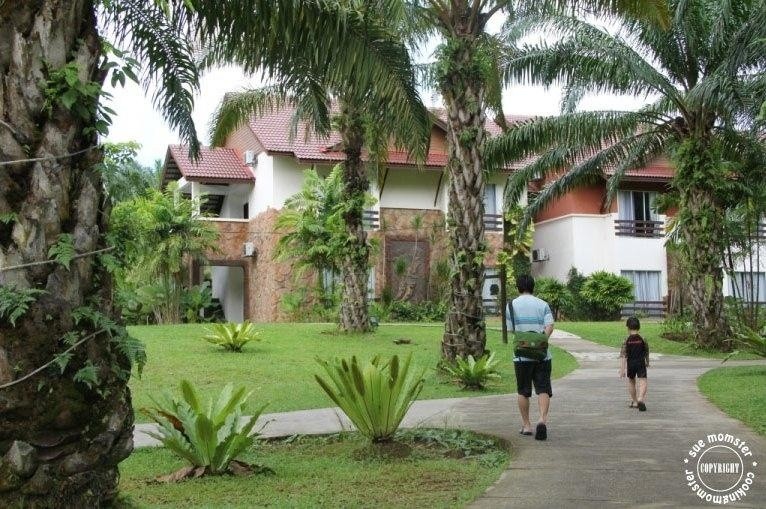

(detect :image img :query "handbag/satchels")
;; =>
[512,332,549,360]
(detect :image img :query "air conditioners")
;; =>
[240,242,254,258]
[242,151,255,167]
[532,248,546,262]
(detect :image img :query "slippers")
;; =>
[535,424,548,440]
[519,425,532,435]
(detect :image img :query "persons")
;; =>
[504,274,555,441]
[617,317,650,412]
